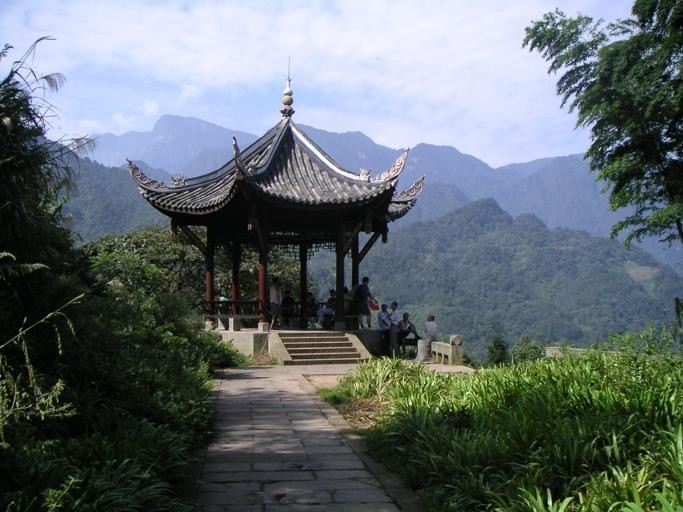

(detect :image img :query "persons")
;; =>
[301,292,316,325]
[280,290,293,324]
[269,278,282,327]
[343,288,351,311]
[421,315,437,340]
[398,312,420,340]
[321,288,336,326]
[377,303,391,356]
[356,277,374,328]
[388,301,401,359]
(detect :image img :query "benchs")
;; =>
[199,300,363,331]
